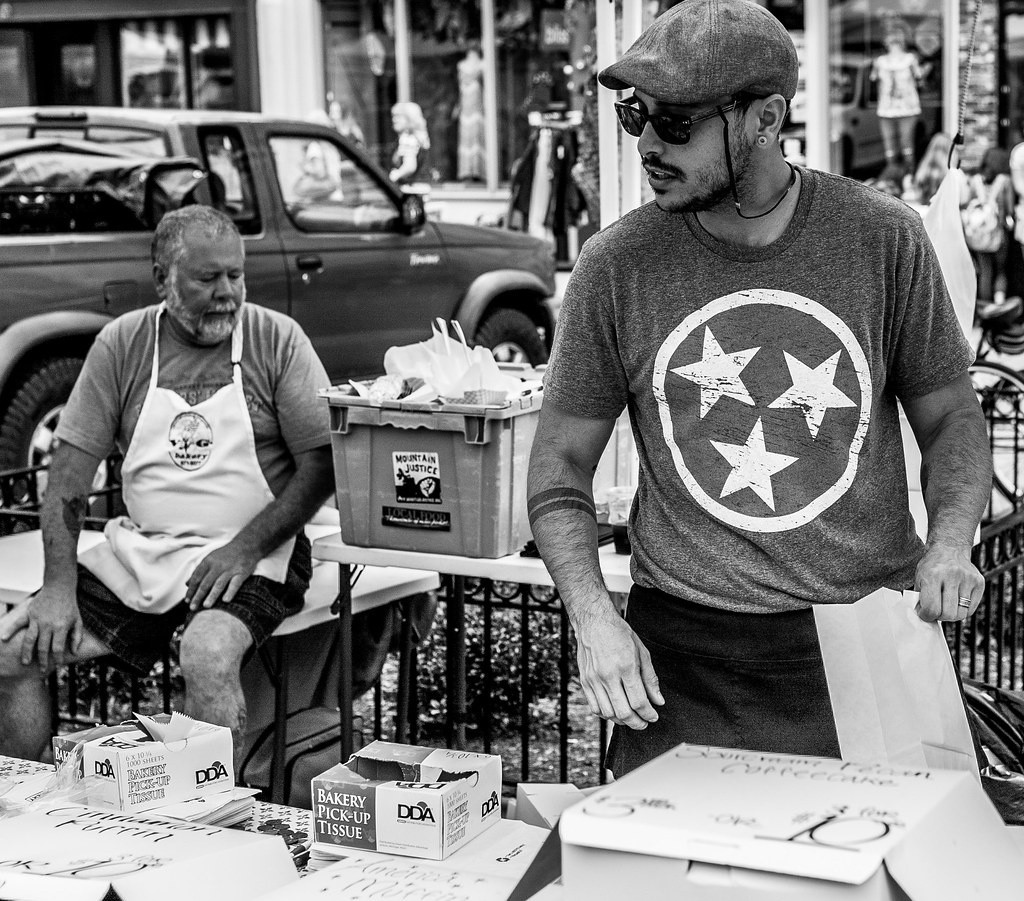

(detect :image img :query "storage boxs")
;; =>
[559,742,973,901]
[311,740,502,861]
[517,783,585,830]
[53,713,235,813]
[316,362,639,559]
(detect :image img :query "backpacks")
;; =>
[961,172,1004,251]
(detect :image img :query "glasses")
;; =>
[614,93,737,145]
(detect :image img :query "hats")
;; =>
[598,0,798,109]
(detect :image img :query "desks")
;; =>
[0,529,440,806]
[313,533,635,765]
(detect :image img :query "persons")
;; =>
[525,0,994,784]
[322,99,367,206]
[452,44,483,181]
[388,101,432,183]
[300,108,345,202]
[984,142,1024,373]
[870,34,933,180]
[0,203,336,784]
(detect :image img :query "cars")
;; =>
[786,55,940,179]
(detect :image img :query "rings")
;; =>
[959,596,971,608]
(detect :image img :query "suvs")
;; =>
[0,98,564,525]
